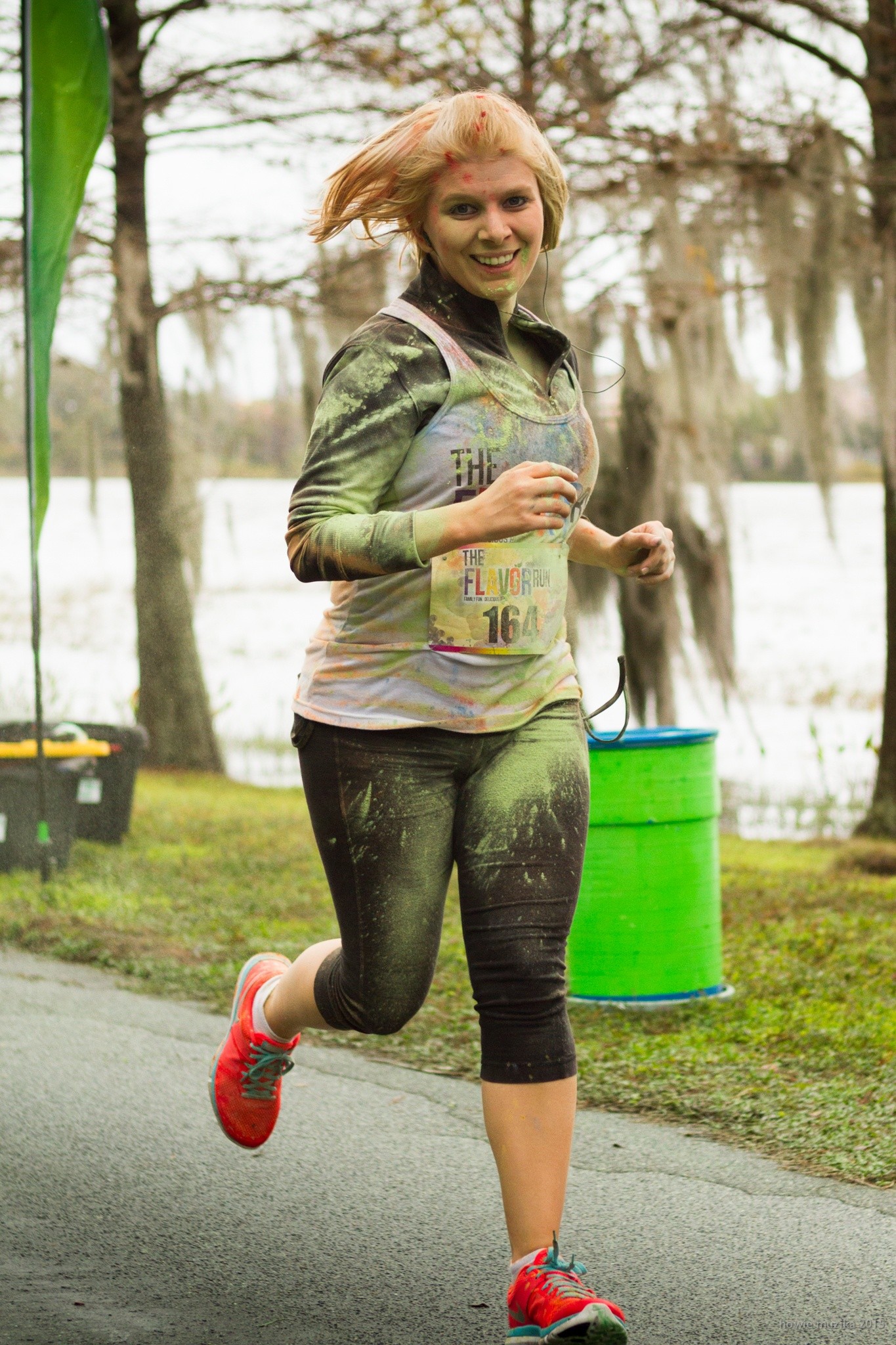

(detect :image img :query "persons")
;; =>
[202,91,674,1345]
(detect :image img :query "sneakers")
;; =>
[209,952,301,1149]
[506,1228,631,1344]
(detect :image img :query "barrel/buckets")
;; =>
[560,728,734,1007]
